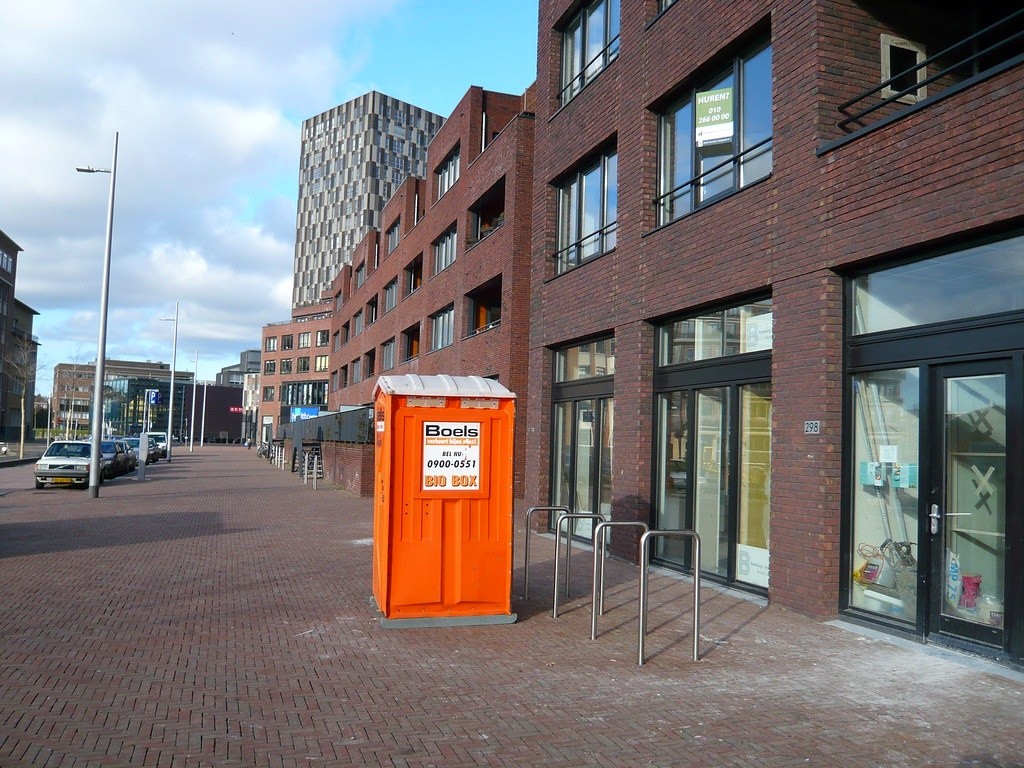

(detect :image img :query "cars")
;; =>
[117,441,136,472]
[120,438,158,466]
[34,440,106,489]
[82,440,129,479]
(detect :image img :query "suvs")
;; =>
[143,432,167,458]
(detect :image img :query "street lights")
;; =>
[186,349,198,452]
[200,373,207,446]
[160,302,179,462]
[75,130,120,493]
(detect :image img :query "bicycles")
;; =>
[256,441,269,458]
